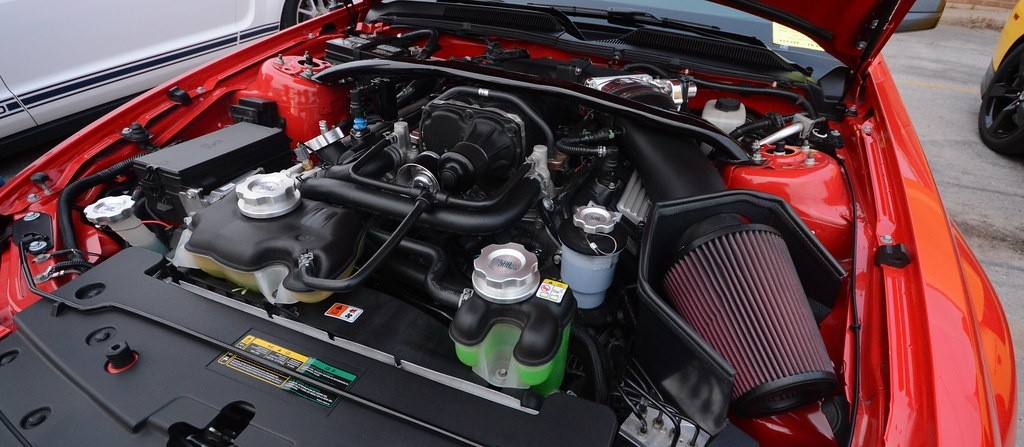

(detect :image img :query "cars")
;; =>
[972,1,1022,156]
[0,0,1017,447]
[0,2,361,177]
